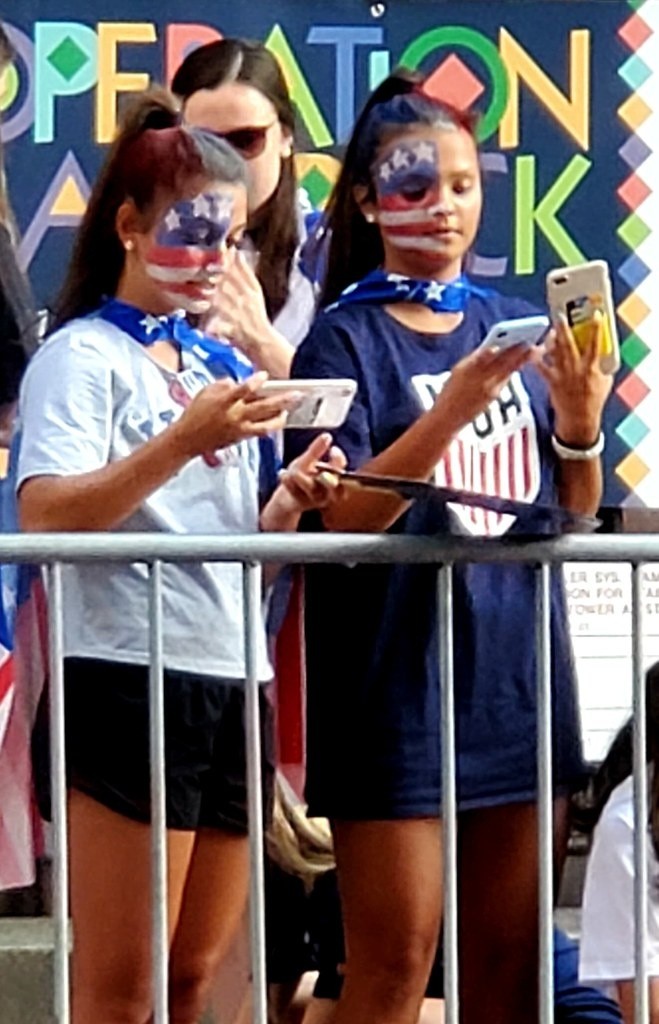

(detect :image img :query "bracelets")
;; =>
[552,429,605,463]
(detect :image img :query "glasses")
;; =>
[221,121,280,159]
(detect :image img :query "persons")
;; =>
[0,39,659,1024]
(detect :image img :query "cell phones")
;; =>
[547,260,620,374]
[477,315,550,353]
[256,380,358,428]
